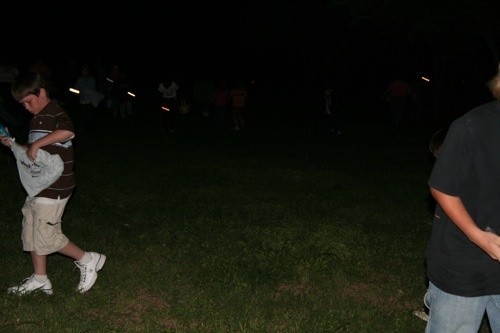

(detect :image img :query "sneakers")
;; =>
[9,272,55,296]
[73,250,106,293]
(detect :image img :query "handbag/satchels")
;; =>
[11,144,69,198]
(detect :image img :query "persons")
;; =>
[421,63,500,333]
[0,54,416,134]
[0,72,107,295]
